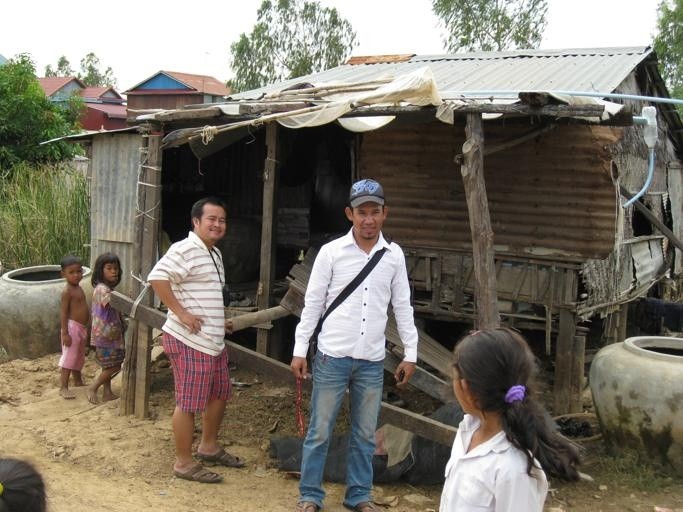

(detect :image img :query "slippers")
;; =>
[342,500,384,512]
[295,502,318,512]
[175,461,224,483]
[198,446,245,467]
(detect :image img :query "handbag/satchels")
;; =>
[305,321,323,363]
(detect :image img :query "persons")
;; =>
[85,253,126,402]
[290,179,418,511]
[0,457,47,511]
[145,197,246,483]
[56,253,89,400]
[436,324,584,511]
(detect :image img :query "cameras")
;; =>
[223,284,245,307]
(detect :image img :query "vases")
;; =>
[0,265,94,361]
[589,337,683,481]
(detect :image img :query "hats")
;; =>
[348,176,387,210]
